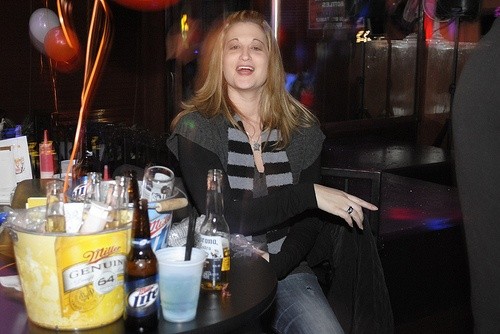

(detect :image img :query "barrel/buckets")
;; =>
[7,201,135,329]
[86,180,189,249]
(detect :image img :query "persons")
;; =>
[166,10,378,334]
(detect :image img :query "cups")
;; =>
[142,165,176,198]
[155,246,206,323]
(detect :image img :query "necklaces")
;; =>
[247,132,261,150]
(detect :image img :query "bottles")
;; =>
[198,169,232,291]
[0,141,139,237]
[124,198,165,333]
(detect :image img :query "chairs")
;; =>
[306,168,383,298]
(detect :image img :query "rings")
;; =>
[347,207,354,213]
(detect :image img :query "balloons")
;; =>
[44,26,80,62]
[30,8,60,42]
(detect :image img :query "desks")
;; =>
[0,223,280,334]
